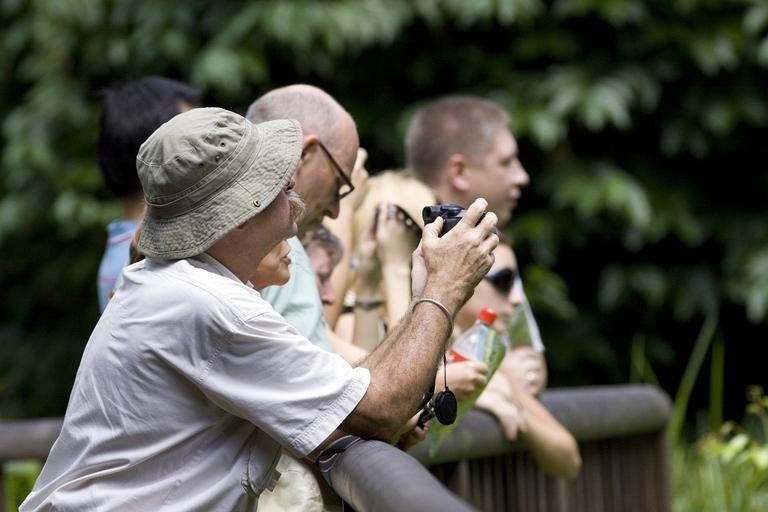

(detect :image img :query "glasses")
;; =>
[320,143,354,202]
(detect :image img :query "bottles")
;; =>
[441,305,500,364]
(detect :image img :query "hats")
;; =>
[132,107,303,261]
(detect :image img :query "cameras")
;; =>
[421,204,485,238]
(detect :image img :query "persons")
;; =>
[235,83,582,511]
[14,103,501,512]
[92,72,203,313]
